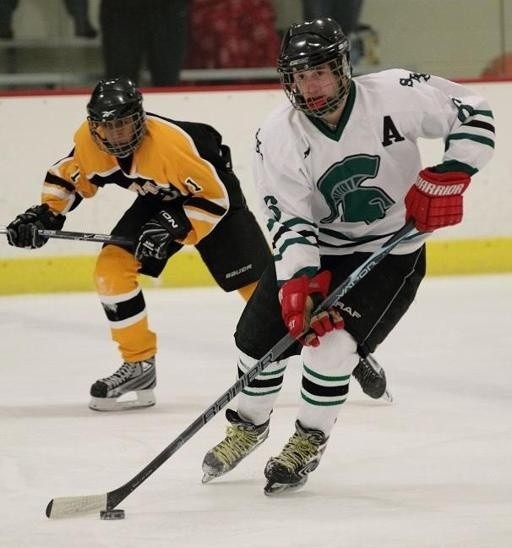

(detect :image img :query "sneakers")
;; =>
[351,354,389,399]
[89,354,158,400]
[74,16,97,39]
[0,18,14,41]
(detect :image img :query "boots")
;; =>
[201,408,274,478]
[263,418,331,485]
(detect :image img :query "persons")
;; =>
[178,0,282,87]
[1,0,97,42]
[202,15,497,486]
[4,75,387,401]
[98,0,186,88]
[302,0,381,66]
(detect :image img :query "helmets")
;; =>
[86,76,144,158]
[277,16,353,115]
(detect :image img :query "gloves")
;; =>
[404,168,472,233]
[6,202,67,250]
[133,205,192,261]
[280,269,345,348]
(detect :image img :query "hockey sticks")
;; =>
[46,217,415,520]
